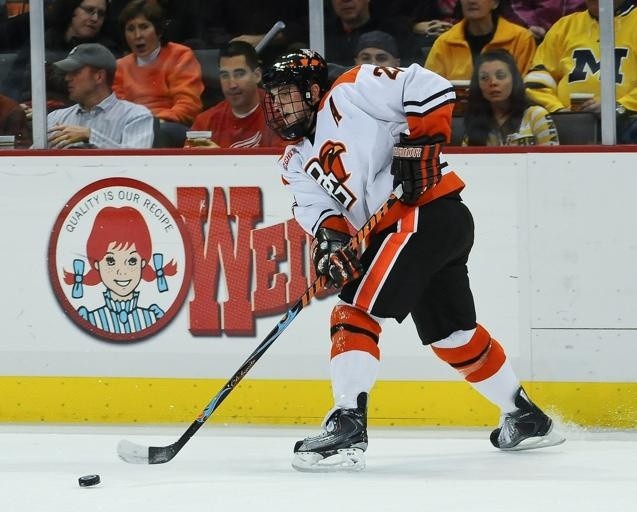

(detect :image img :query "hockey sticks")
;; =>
[116,187,402,463]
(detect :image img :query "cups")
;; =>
[185,131,211,147]
[570,93,594,112]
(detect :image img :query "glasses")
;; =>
[76,4,106,18]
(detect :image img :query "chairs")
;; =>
[0,20,637,147]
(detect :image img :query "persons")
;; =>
[255,48,554,456]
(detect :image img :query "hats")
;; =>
[51,42,116,74]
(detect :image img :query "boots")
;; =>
[291,387,372,458]
[488,386,557,452]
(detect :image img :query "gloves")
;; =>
[310,226,366,290]
[387,131,449,206]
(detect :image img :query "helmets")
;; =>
[255,46,330,105]
